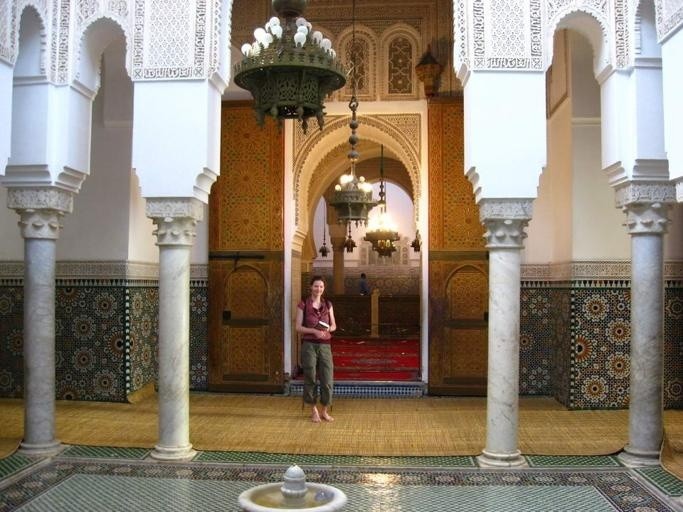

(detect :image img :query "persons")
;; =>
[295,275,338,424]
[359,272,370,296]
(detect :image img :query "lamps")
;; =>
[231,2,346,135]
[319,0,443,258]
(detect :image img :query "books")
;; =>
[317,321,329,331]
